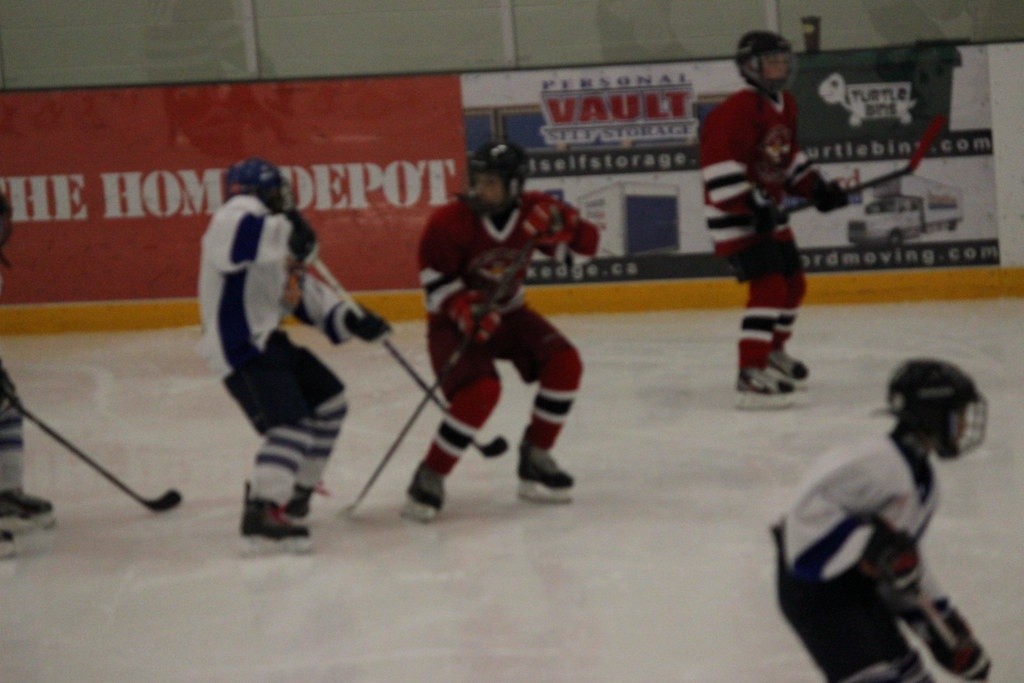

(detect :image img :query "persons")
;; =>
[397,140,600,524]
[695,28,851,411]
[198,156,389,555]
[768,357,992,683]
[0,360,56,559]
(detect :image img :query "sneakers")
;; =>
[286,481,319,519]
[736,368,798,409]
[397,460,445,522]
[0,528,16,559]
[517,446,573,501]
[766,348,809,385]
[0,489,55,530]
[239,482,314,549]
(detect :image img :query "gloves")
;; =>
[879,534,922,596]
[749,190,781,235]
[281,207,317,265]
[445,289,501,340]
[344,307,391,342]
[808,176,849,212]
[927,609,992,682]
[523,199,580,244]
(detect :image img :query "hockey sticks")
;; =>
[1,389,182,513]
[342,234,542,514]
[311,259,511,460]
[785,113,948,215]
[917,592,989,682]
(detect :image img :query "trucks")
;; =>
[848,173,963,248]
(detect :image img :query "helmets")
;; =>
[468,141,528,190]
[221,156,291,214]
[736,29,798,91]
[886,359,990,461]
